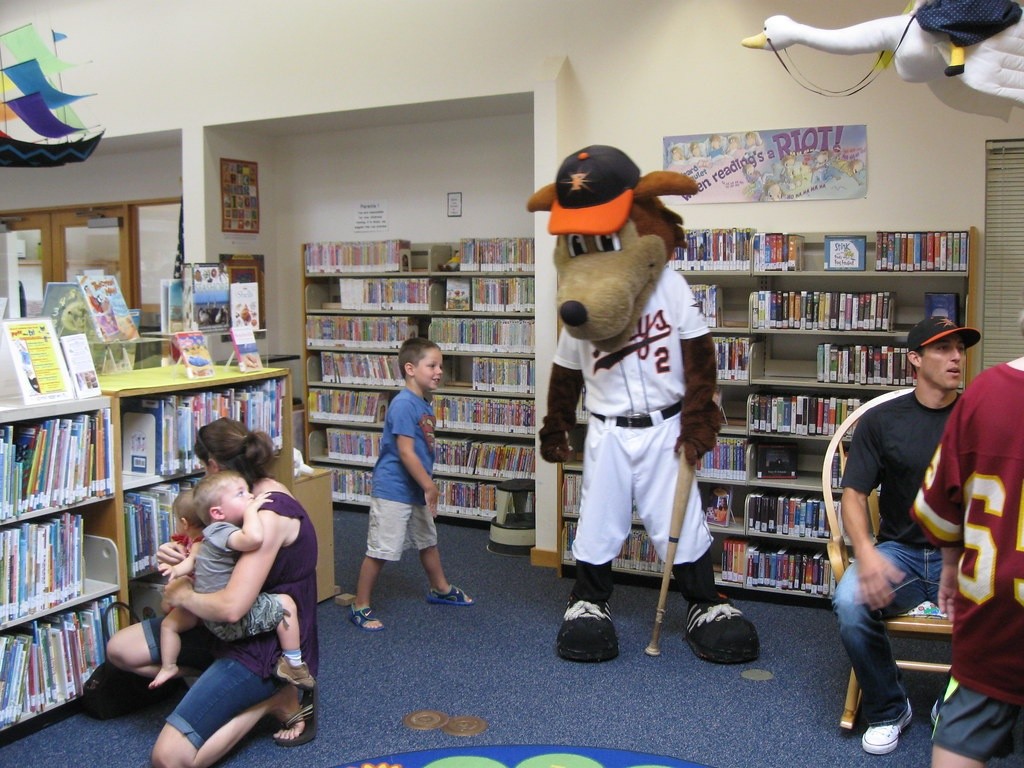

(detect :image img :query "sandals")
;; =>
[348,603,385,631]
[426,584,474,606]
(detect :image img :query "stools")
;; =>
[486,479,536,557]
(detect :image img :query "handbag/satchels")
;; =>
[83,602,191,719]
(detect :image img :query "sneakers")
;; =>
[862,698,913,755]
[930,699,938,725]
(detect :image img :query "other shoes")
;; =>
[272,654,317,690]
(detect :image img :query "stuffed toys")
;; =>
[527,144,759,664]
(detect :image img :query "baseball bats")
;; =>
[643,451,695,657]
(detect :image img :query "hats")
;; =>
[907,316,981,350]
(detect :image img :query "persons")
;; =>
[194,471,316,688]
[106,416,319,768]
[909,311,1024,768]
[831,317,982,754]
[349,337,474,631]
[148,492,206,688]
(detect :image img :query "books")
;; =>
[0,262,282,728]
[561,228,967,617]
[304,237,536,521]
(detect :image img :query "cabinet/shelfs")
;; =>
[0,241,982,744]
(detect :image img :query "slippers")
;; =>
[275,685,319,747]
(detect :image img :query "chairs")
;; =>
[821,388,954,730]
